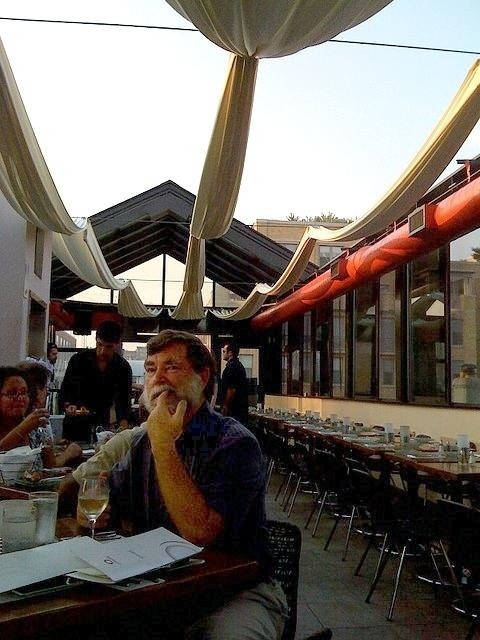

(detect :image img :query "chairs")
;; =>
[0,390,480,640]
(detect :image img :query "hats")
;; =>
[47,343,56,348]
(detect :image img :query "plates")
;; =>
[0,450,38,487]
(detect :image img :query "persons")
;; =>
[52,420,151,494]
[47,342,58,364]
[18,358,84,469]
[217,341,249,427]
[0,364,51,450]
[58,320,134,443]
[94,331,291,640]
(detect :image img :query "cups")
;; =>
[0,498,37,553]
[254,403,471,467]
[28,490,60,546]
[31,407,52,435]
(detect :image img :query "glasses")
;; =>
[0,389,27,398]
[222,351,229,353]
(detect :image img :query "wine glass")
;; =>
[76,476,110,540]
